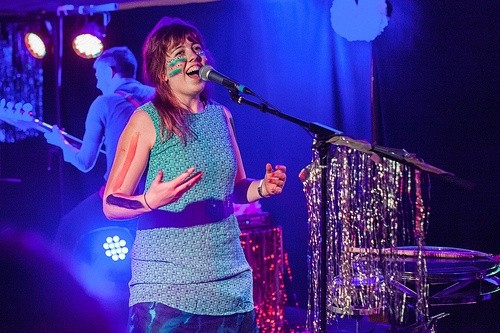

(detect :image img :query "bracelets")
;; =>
[144,191,155,211]
[60,143,70,148]
[258,179,272,198]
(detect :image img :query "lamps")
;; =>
[24,29,58,59]
[72,20,107,60]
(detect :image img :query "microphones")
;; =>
[199,64,255,96]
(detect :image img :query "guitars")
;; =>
[0,97,108,168]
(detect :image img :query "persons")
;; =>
[103,16,287,333]
[44,47,157,253]
